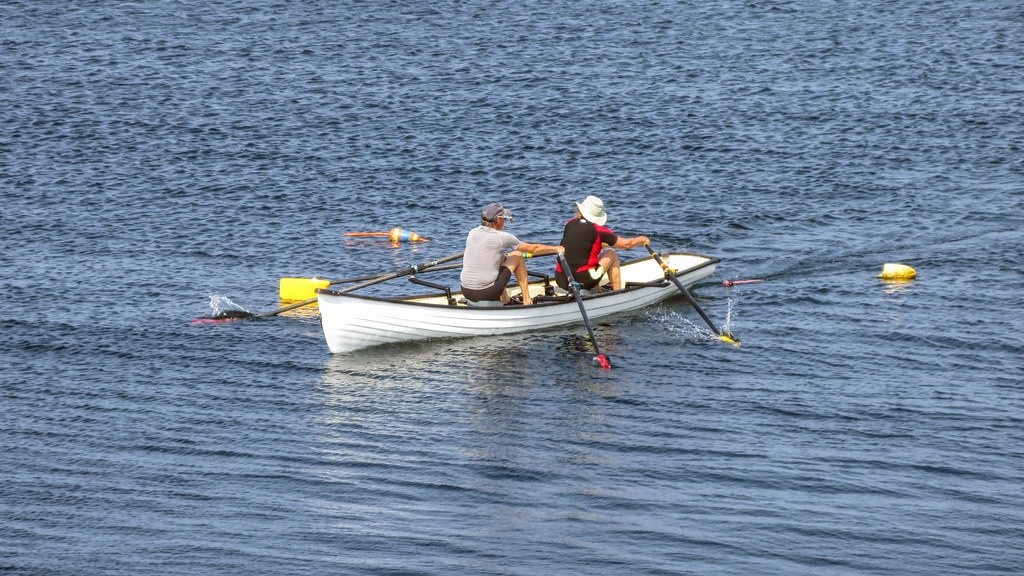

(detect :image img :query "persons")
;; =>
[459,203,566,306]
[554,195,652,290]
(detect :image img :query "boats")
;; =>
[314,252,721,353]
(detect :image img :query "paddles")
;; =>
[642,242,742,349]
[623,279,765,287]
[191,252,465,326]
[278,251,559,303]
[558,251,614,370]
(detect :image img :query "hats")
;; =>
[483,202,513,222]
[575,195,607,226]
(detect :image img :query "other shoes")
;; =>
[503,297,516,307]
